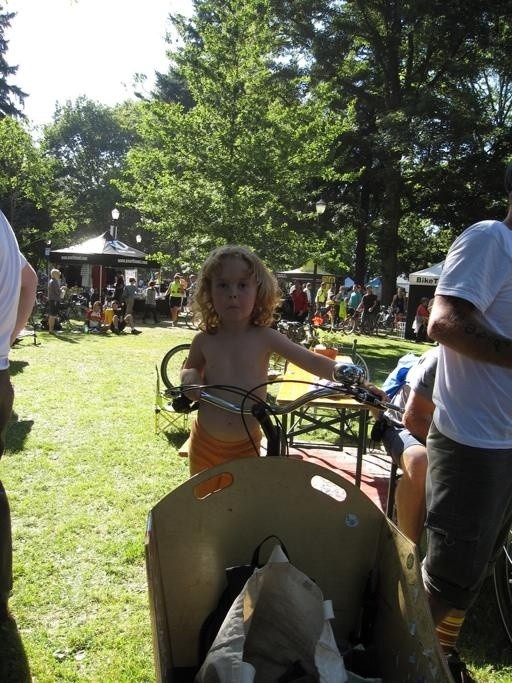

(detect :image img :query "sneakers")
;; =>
[131,329,142,334]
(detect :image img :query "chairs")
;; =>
[143,456,457,683]
[153,363,188,436]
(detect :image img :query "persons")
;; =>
[412,296,429,342]
[271,278,378,334]
[179,245,390,499]
[382,345,441,551]
[390,288,406,332]
[0,210,40,612]
[421,201,511,683]
[48,268,198,336]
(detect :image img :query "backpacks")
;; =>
[381,353,420,399]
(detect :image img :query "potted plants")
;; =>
[313,328,344,360]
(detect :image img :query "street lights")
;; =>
[112,206,120,239]
[136,234,143,249]
[309,194,328,305]
[46,239,52,297]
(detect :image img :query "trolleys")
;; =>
[143,364,456,682]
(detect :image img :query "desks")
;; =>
[276,355,370,489]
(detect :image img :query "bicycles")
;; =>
[185,303,399,338]
[29,281,93,331]
[277,320,370,396]
